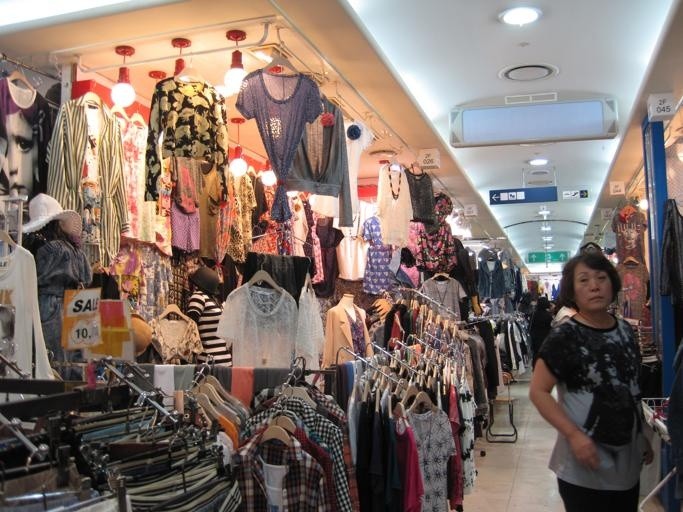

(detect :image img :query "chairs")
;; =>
[485,372,517,443]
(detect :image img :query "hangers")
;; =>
[262,26,403,173]
[129,103,145,126]
[8,59,34,94]
[622,251,638,264]
[0,211,18,250]
[0,338,323,512]
[109,104,127,119]
[351,287,467,430]
[249,251,280,291]
[406,160,448,281]
[81,80,98,106]
[148,297,192,326]
[622,199,636,213]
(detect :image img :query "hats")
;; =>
[130,313,154,356]
[22,194,82,237]
[189,266,220,295]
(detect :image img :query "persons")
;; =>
[528,296,554,371]
[0,110,38,196]
[527,247,656,512]
[518,280,539,311]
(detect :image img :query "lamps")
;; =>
[148,71,163,146]
[222,30,249,93]
[168,38,190,80]
[228,119,246,176]
[261,159,276,185]
[110,46,135,106]
